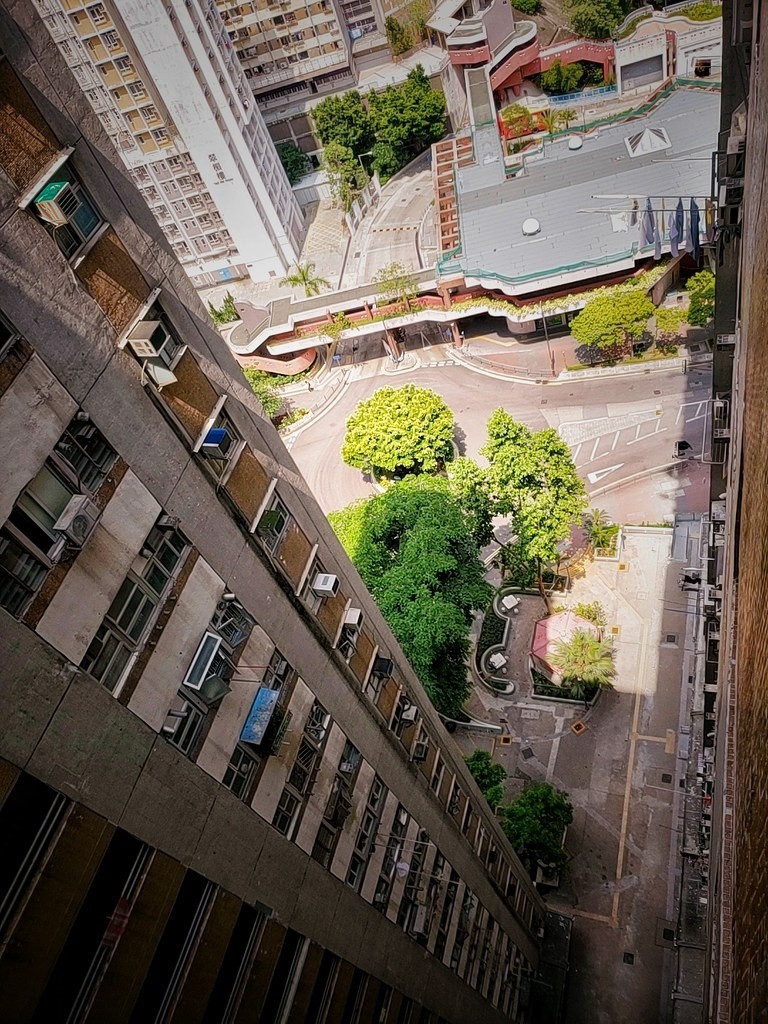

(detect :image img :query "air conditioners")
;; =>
[313,574,340,597]
[263,68,271,75]
[415,741,429,759]
[201,427,238,459]
[403,705,420,724]
[281,62,288,69]
[258,510,286,537]
[35,182,83,225]
[128,320,171,357]
[414,905,431,937]
[52,494,104,549]
[345,608,365,631]
[373,657,395,678]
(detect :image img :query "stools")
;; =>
[487,654,510,674]
[500,596,522,615]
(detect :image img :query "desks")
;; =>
[501,595,519,609]
[489,653,507,670]
